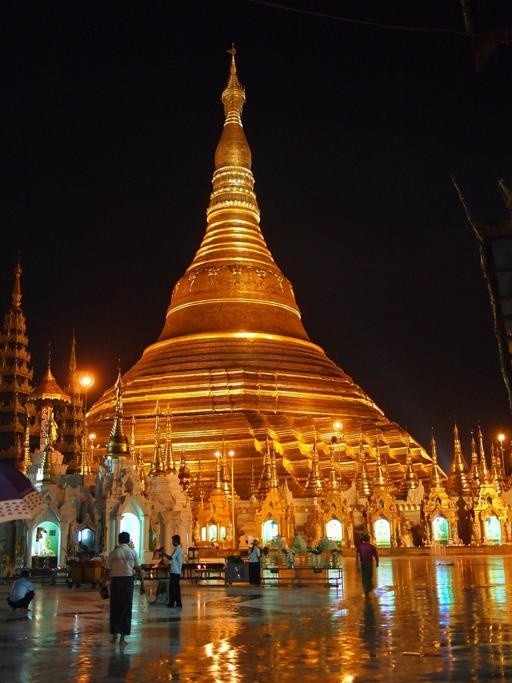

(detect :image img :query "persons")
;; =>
[37,526,48,555]
[169,607,182,682]
[107,644,131,682]
[249,586,263,617]
[157,533,185,608]
[362,594,376,658]
[356,533,380,595]
[248,539,261,586]
[100,531,146,645]
[7,568,36,612]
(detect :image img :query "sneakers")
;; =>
[166,604,182,609]
[110,635,128,645]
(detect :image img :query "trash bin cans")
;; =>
[225,555,249,587]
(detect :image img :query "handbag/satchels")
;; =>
[99,583,109,600]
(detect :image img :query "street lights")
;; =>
[228,449,235,551]
[332,420,344,489]
[88,431,95,465]
[497,433,506,476]
[80,372,94,475]
[214,450,221,495]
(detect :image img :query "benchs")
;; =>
[260,567,343,580]
[194,569,227,585]
